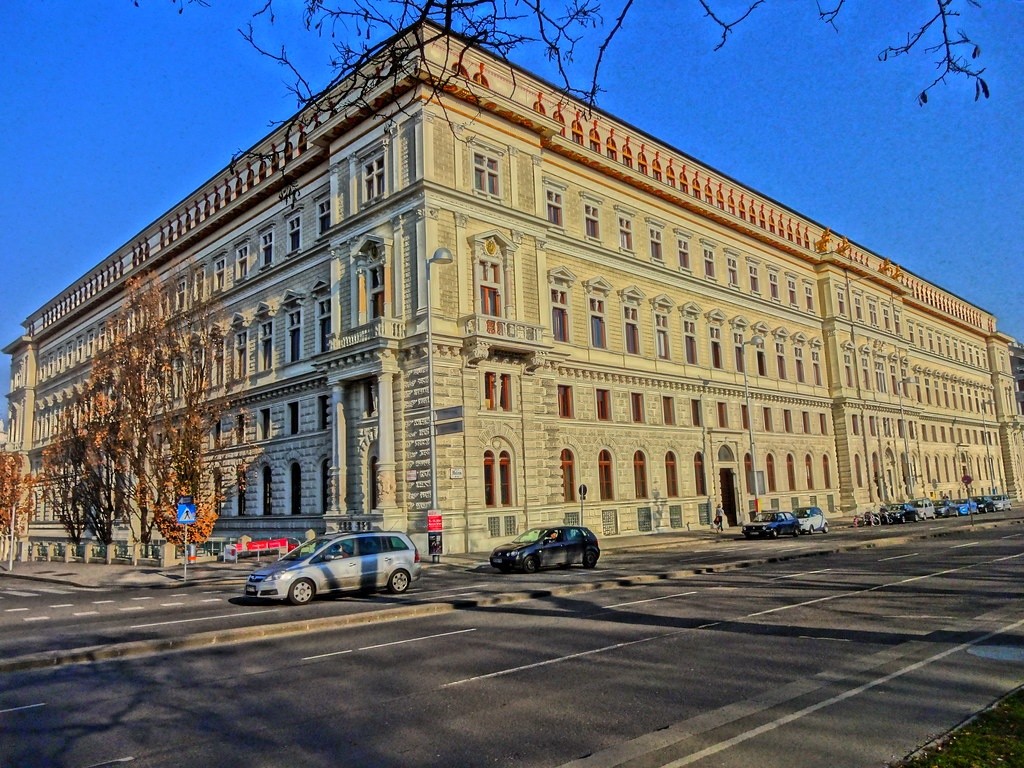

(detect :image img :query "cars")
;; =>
[742,511,802,538]
[992,495,1013,512]
[880,503,920,525]
[931,500,960,519]
[908,498,937,520]
[954,499,980,515]
[971,496,997,514]
[793,507,829,535]
[490,525,600,572]
[244,529,421,605]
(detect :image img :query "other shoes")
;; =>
[721,529,723,531]
[717,527,719,530]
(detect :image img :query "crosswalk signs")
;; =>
[178,506,195,523]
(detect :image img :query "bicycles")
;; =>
[855,510,881,527]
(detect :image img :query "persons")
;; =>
[943,493,948,499]
[548,532,558,544]
[880,505,890,515]
[715,504,724,533]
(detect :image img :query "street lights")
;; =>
[428,248,454,560]
[743,335,763,514]
[898,377,916,499]
[981,400,997,494]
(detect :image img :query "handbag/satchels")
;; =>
[714,517,718,524]
